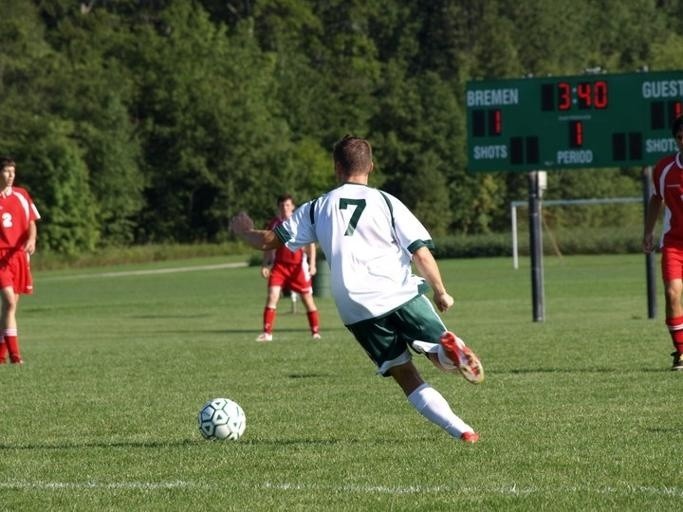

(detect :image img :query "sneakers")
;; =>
[254,333,273,341]
[461,431,478,443]
[671,351,682,370]
[439,330,485,385]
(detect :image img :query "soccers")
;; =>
[198,398,246,441]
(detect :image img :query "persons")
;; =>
[639,114,683,372]
[253,193,323,344]
[226,134,486,445]
[0,156,42,364]
